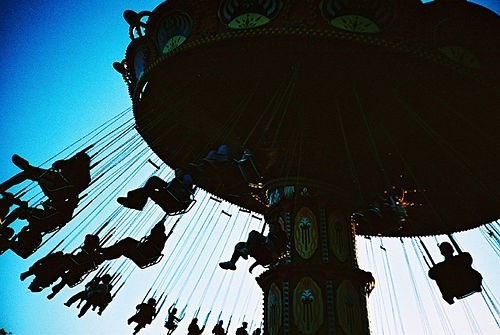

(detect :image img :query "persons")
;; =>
[0,190,112,318]
[428,242,483,305]
[212,320,227,335]
[164,304,183,335]
[187,317,205,335]
[12,151,91,201]
[117,167,192,214]
[219,221,288,270]
[128,298,156,335]
[236,322,248,335]
[253,328,261,335]
[97,222,167,266]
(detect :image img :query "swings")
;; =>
[0,101,499,335]
